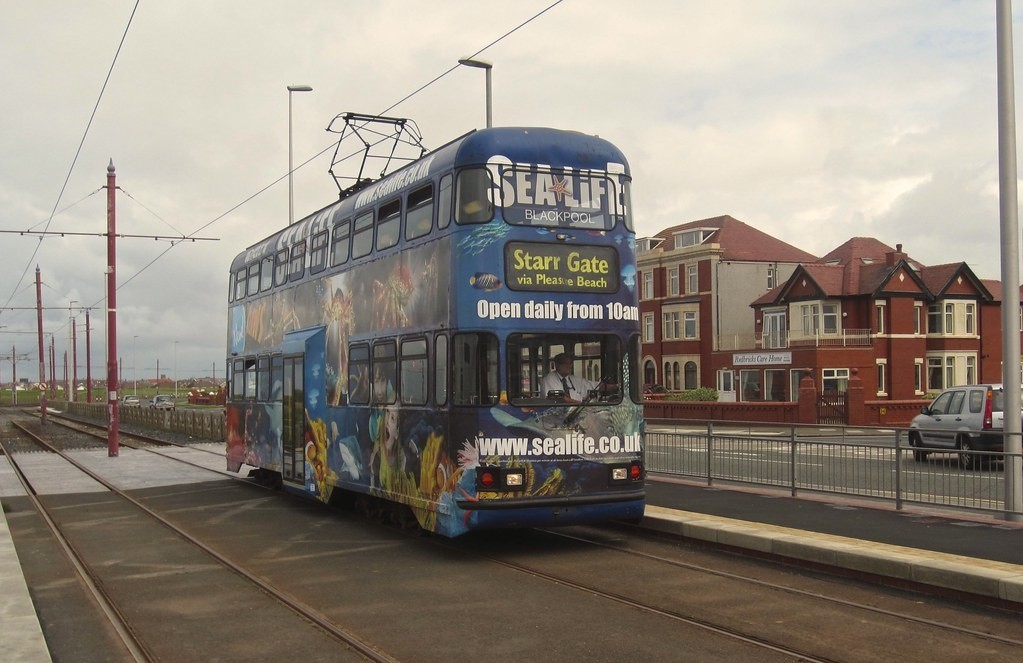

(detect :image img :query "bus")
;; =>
[223,126,647,539]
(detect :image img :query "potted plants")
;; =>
[744,382,759,399]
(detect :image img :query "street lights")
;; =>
[457,55,493,127]
[286,83,313,224]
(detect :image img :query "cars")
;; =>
[907,383,1023,467]
[120,395,141,408]
[149,395,175,411]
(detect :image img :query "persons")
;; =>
[539,354,621,403]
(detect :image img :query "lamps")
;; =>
[757,319,761,324]
[842,311,848,318]
